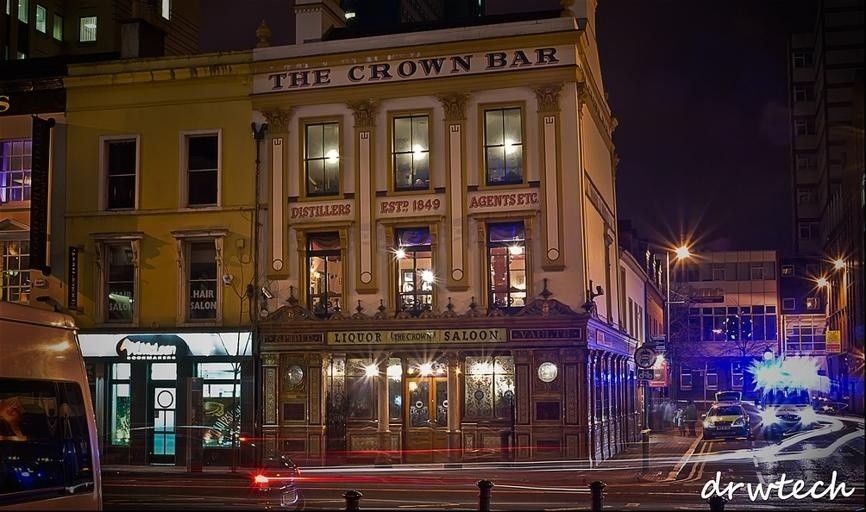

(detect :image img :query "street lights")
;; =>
[252,120,268,468]
[667,245,688,342]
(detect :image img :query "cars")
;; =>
[99,426,305,512]
[761,386,812,429]
[703,391,750,440]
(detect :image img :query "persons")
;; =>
[683,398,698,438]
[674,403,685,437]
[663,400,676,426]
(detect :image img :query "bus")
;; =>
[0,301,103,512]
